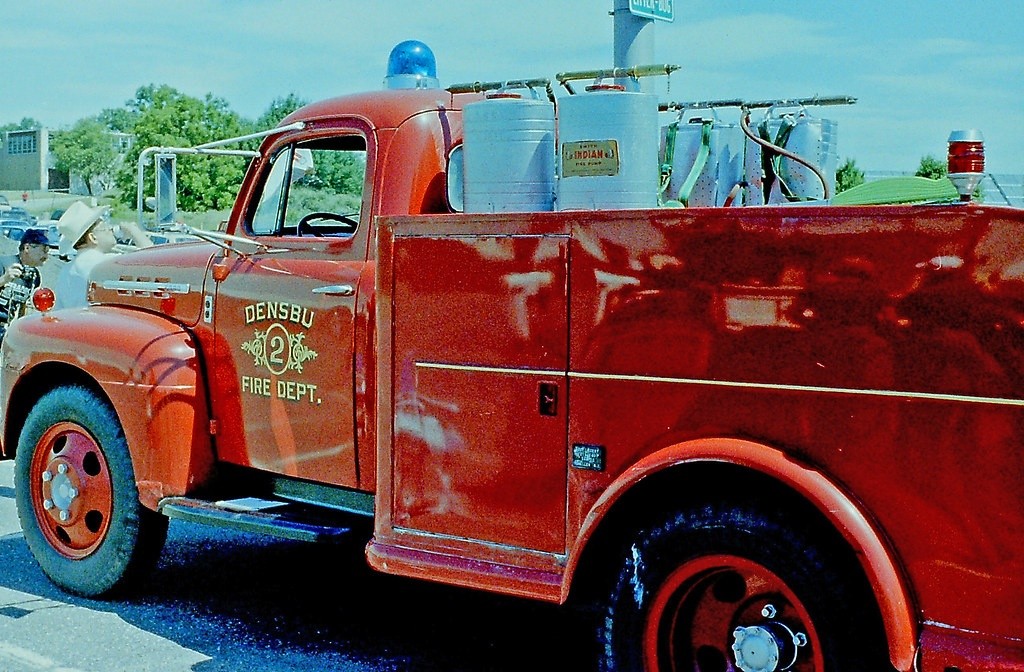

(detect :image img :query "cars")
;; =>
[1,196,33,240]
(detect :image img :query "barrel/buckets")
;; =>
[462,94,555,212]
[660,114,840,206]
[556,85,659,211]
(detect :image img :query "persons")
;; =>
[1,228,49,362]
[56,201,154,309]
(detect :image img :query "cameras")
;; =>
[18,265,36,281]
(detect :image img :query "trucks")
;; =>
[0,40,1024,672]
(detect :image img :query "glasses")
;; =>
[29,244,49,253]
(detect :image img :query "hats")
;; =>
[56,201,111,256]
[21,229,50,246]
